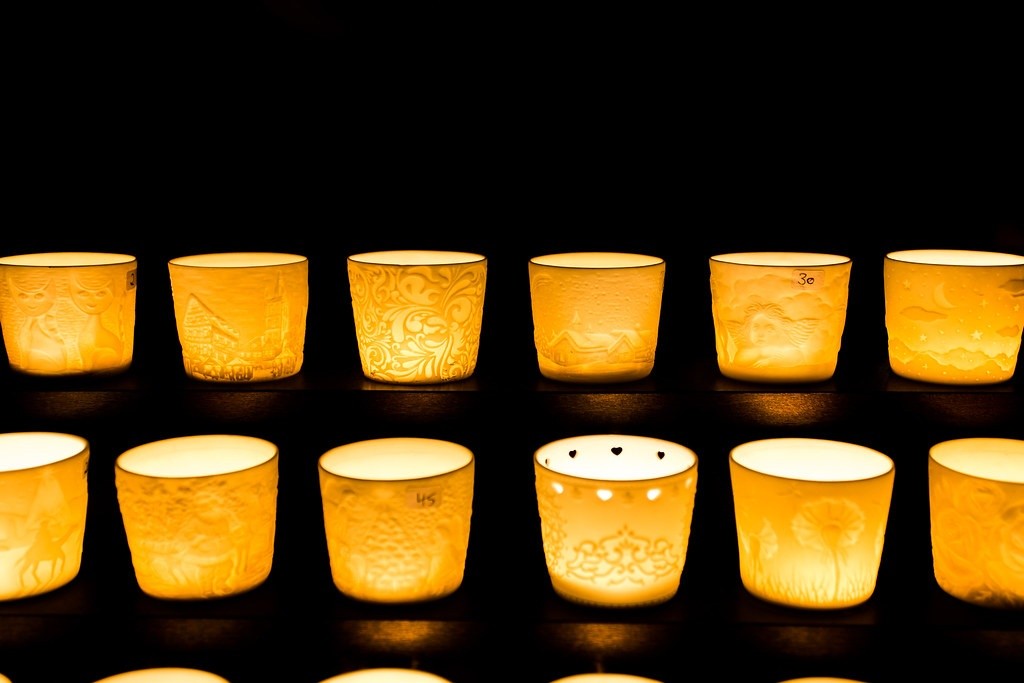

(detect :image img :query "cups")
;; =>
[345,250,492,381]
[881,246,1023,388]
[527,248,667,382]
[728,439,898,611]
[709,248,856,382]
[318,438,476,606]
[115,434,279,602]
[0,430,91,604]
[167,251,311,384]
[924,435,1023,610]
[0,253,138,376]
